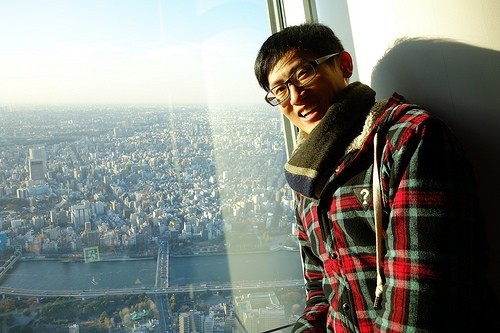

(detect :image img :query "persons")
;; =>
[254,24,436,333]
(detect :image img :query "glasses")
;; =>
[265,52,339,107]
